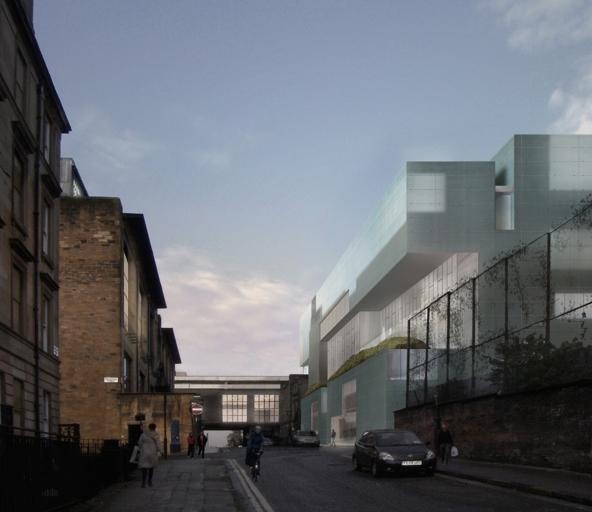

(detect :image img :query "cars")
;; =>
[353,428,437,478]
[292,430,320,448]
[263,437,273,446]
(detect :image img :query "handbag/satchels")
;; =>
[129,446,141,463]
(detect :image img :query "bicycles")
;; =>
[245,449,264,484]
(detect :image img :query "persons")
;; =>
[244,424,265,475]
[136,423,164,488]
[329,428,337,447]
[196,430,208,458]
[437,421,455,466]
[186,431,196,457]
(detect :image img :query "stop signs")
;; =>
[192,403,204,415]
